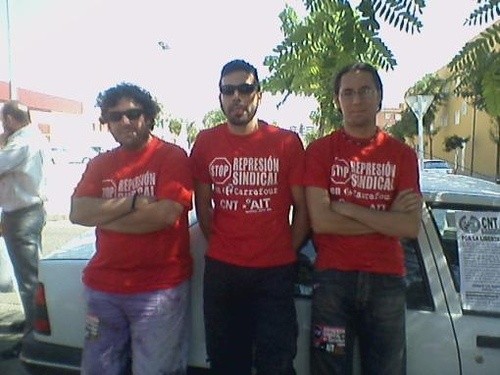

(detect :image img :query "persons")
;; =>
[69,82,196,375]
[0,103,47,358]
[303,61,423,375]
[189,60,310,375]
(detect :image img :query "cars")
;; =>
[19,160,500,374]
[50,144,99,164]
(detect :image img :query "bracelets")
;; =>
[131,193,138,211]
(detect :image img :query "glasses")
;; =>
[104,109,144,122]
[220,83,256,95]
[340,88,378,98]
